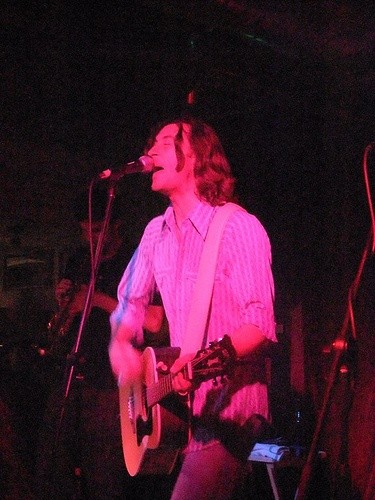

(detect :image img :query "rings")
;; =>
[179,391,189,396]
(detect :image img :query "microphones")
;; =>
[98,155,154,179]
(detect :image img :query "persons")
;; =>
[40,188,135,500]
[107,117,279,500]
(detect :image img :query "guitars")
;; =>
[119,332,236,478]
